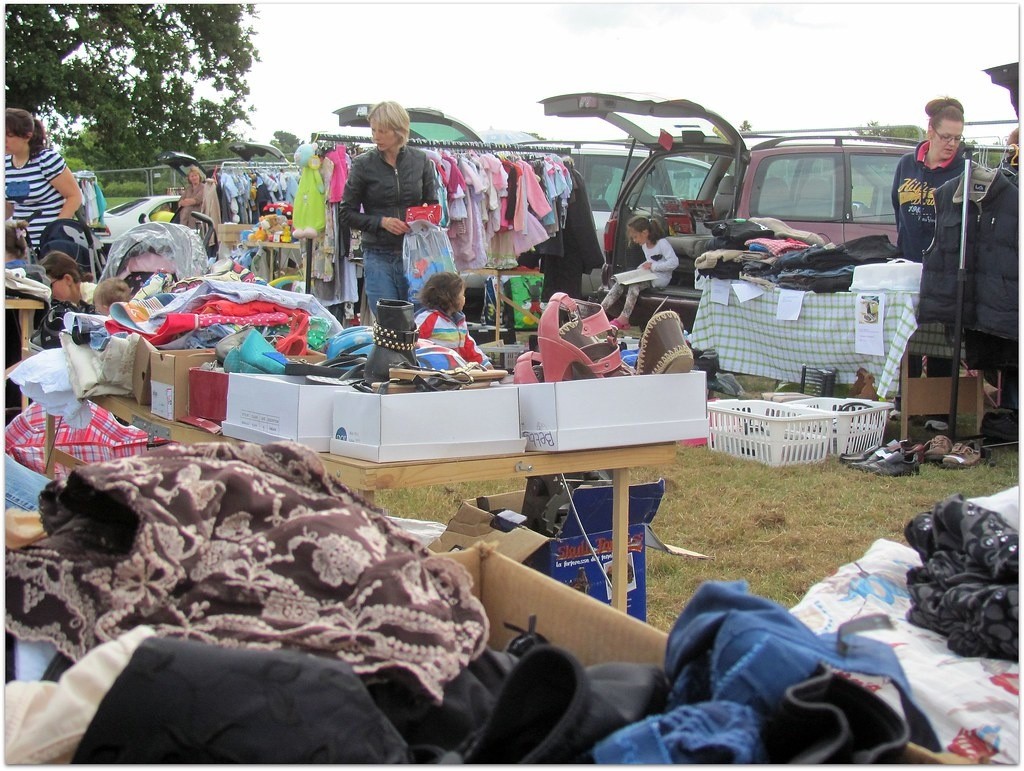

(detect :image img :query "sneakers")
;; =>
[981,412,1019,441]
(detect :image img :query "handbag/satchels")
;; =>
[401,227,458,306]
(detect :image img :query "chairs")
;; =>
[588,173,615,212]
[712,176,736,219]
[800,177,830,199]
[761,176,791,198]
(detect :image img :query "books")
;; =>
[611,269,659,285]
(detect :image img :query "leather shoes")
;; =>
[838,435,981,478]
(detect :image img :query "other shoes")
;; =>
[621,311,694,374]
[500,292,627,384]
[889,410,910,420]
[925,420,948,431]
[609,318,630,330]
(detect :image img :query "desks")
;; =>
[692,278,982,441]
[245,241,306,284]
[45,394,678,615]
[5,299,44,414]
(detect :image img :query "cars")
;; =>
[99,142,301,266]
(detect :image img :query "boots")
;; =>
[364,298,420,384]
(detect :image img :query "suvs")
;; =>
[330,103,735,299]
[537,89,1008,364]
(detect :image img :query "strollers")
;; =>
[22,209,111,359]
[92,210,220,322]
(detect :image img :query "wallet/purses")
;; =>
[405,204,442,226]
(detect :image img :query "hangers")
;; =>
[223,161,300,173]
[968,144,1019,176]
[167,188,186,192]
[71,177,95,184]
[311,132,573,154]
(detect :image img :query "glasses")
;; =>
[49,279,58,290]
[930,124,965,144]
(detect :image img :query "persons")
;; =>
[601,216,680,329]
[339,101,440,320]
[5,220,51,288]
[93,277,129,316]
[178,167,205,228]
[413,271,494,370]
[4,109,82,255]
[38,251,98,307]
[889,99,973,431]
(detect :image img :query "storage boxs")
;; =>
[433,469,671,667]
[131,334,706,462]
[707,393,895,467]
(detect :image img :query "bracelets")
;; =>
[384,216,392,228]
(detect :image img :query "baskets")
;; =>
[707,396,895,468]
